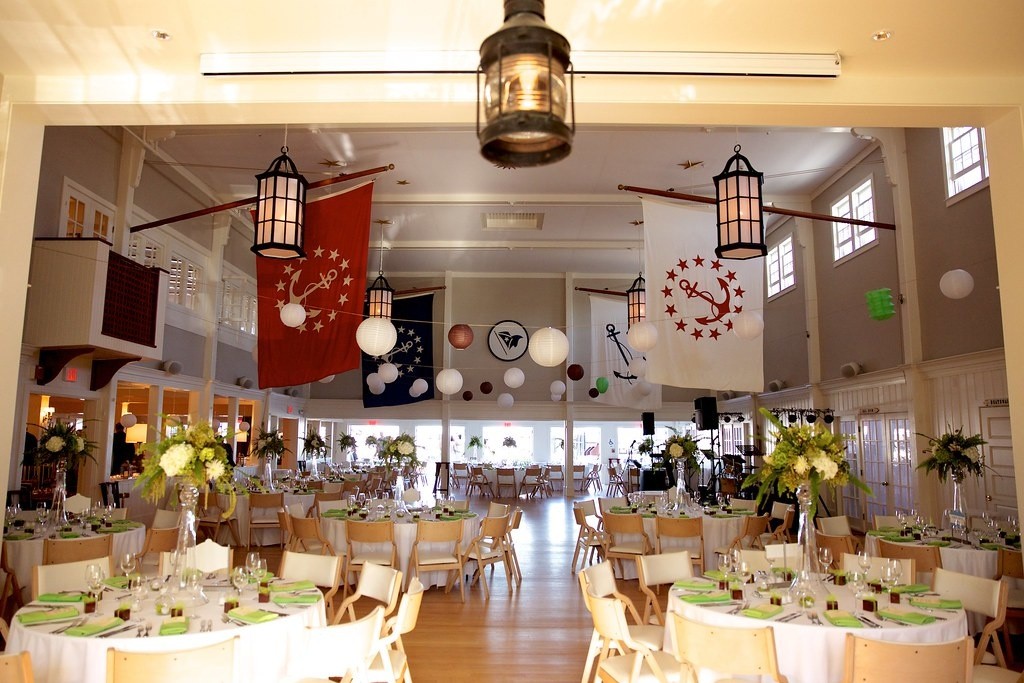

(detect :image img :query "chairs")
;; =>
[837,551,916,585]
[815,514,862,554]
[1,454,793,683]
[984,548,1024,664]
[876,539,944,572]
[814,529,854,571]
[871,513,916,533]
[930,565,1008,670]
[969,515,999,530]
[842,634,974,683]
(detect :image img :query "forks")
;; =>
[221,614,250,627]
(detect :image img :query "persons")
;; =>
[215,435,235,469]
[111,422,135,475]
[24,425,38,466]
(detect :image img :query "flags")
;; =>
[641,201,765,391]
[359,293,436,409]
[588,295,662,409]
[257,180,373,388]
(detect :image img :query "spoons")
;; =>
[49,618,89,635]
[135,624,152,638]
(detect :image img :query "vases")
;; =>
[308,461,321,483]
[472,443,477,464]
[44,473,72,532]
[673,458,692,513]
[263,462,275,493]
[394,470,406,506]
[152,480,210,607]
[951,474,969,514]
[786,485,831,604]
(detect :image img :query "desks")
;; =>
[453,462,482,488]
[471,464,540,497]
[663,570,968,683]
[863,531,1024,635]
[606,503,759,575]
[214,487,315,548]
[319,508,480,592]
[543,462,589,491]
[1,512,145,589]
[5,573,328,682]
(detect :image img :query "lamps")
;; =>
[249,144,309,262]
[625,218,645,335]
[712,144,768,263]
[365,218,393,320]
[473,1,578,170]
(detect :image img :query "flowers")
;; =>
[339,433,357,453]
[252,429,287,461]
[378,437,418,469]
[661,431,699,465]
[141,414,233,497]
[915,427,990,483]
[761,411,854,496]
[31,425,91,468]
[467,434,483,449]
[301,433,330,458]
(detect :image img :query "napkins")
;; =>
[4,505,1022,635]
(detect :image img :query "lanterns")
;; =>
[448,323,474,350]
[627,319,658,396]
[497,393,514,410]
[356,316,397,357]
[436,367,463,394]
[367,362,398,395]
[529,327,570,368]
[280,302,306,327]
[463,390,473,401]
[479,382,493,394]
[407,378,428,397]
[551,364,609,403]
[504,367,526,389]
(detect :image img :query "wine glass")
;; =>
[5,500,116,534]
[83,551,268,621]
[246,477,308,493]
[628,490,735,517]
[891,509,1020,543]
[346,492,455,521]
[718,548,902,611]
